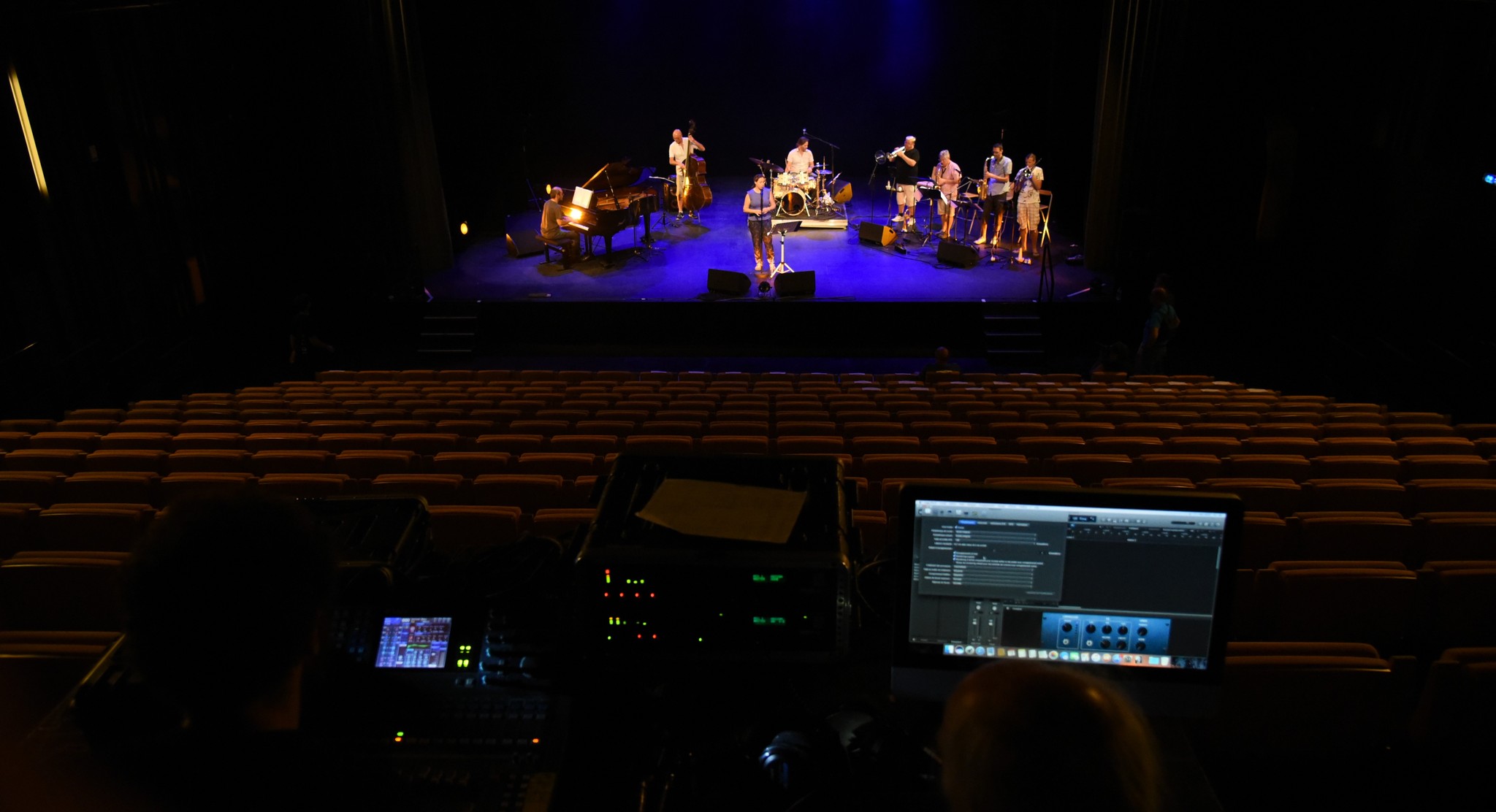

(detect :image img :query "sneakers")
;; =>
[907,217,916,225]
[891,215,904,222]
[688,212,698,218]
[675,212,684,220]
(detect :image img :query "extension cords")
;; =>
[989,259,1001,263]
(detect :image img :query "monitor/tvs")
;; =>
[889,484,1243,693]
[377,615,454,671]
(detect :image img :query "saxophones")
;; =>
[977,155,996,201]
[935,163,943,201]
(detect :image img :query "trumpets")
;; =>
[900,208,911,233]
[874,146,906,165]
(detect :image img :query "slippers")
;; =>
[1013,248,1027,253]
[1033,250,1039,256]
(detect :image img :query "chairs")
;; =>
[950,180,1052,247]
[0,368,1496,812]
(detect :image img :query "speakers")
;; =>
[825,179,853,204]
[937,241,980,270]
[858,221,898,247]
[707,269,751,295]
[774,270,816,297]
[506,228,544,257]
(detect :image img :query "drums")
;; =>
[804,179,817,194]
[773,177,787,197]
[777,172,793,187]
[780,187,806,216]
[795,171,809,186]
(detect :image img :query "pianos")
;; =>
[559,162,661,269]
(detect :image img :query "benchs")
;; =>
[535,234,573,271]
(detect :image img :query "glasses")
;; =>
[939,158,948,162]
[673,135,682,140]
[993,151,1001,154]
[904,141,913,145]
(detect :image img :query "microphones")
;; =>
[803,128,806,135]
[954,168,962,174]
[967,177,980,184]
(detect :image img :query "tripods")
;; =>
[898,177,1031,269]
[619,177,679,262]
[806,133,844,217]
[766,220,802,280]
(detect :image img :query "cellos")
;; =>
[681,119,713,225]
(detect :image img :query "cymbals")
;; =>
[813,164,829,167]
[749,157,784,173]
[815,170,832,174]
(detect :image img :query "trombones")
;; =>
[1008,157,1043,190]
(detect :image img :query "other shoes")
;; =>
[580,248,583,253]
[769,264,776,275]
[973,237,986,244]
[578,255,590,263]
[935,230,944,236]
[938,232,950,238]
[755,263,762,271]
[990,238,999,245]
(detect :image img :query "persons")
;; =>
[743,174,776,271]
[974,144,1012,245]
[889,136,920,225]
[1012,153,1044,257]
[936,660,1160,812]
[541,187,590,263]
[785,137,821,212]
[669,129,705,220]
[934,150,962,238]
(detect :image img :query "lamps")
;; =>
[758,281,772,298]
